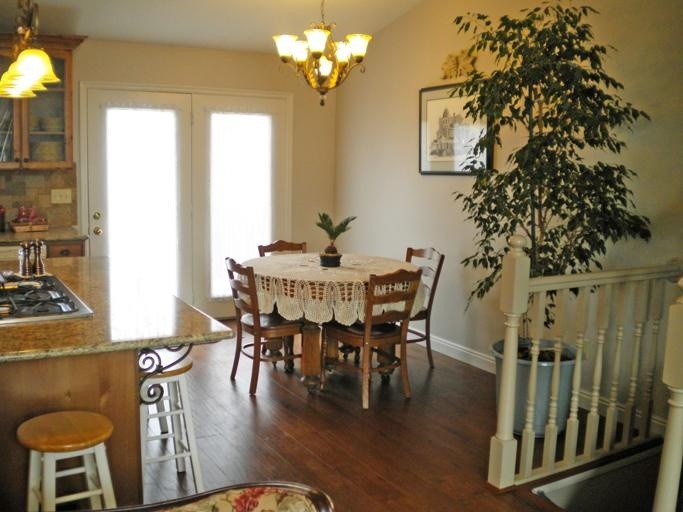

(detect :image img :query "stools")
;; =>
[17,412,116,510]
[138,349,205,494]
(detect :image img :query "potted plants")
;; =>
[313,210,355,268]
[442,1,650,441]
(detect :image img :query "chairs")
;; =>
[397,246,443,369]
[323,268,420,409]
[257,241,307,356]
[224,254,301,394]
[92,480,332,511]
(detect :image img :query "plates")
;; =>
[28,118,62,132]
[8,220,48,232]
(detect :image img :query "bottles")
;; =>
[18,240,31,275]
[0,204,6,233]
[32,239,43,276]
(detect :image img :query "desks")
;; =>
[235,255,415,397]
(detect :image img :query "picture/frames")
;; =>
[414,79,493,177]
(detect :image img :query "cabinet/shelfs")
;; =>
[0,29,86,171]
[42,238,85,261]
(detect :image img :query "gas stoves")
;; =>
[0,274,93,327]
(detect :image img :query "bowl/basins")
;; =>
[31,141,65,162]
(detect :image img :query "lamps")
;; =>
[272,1,374,106]
[0,0,63,101]
